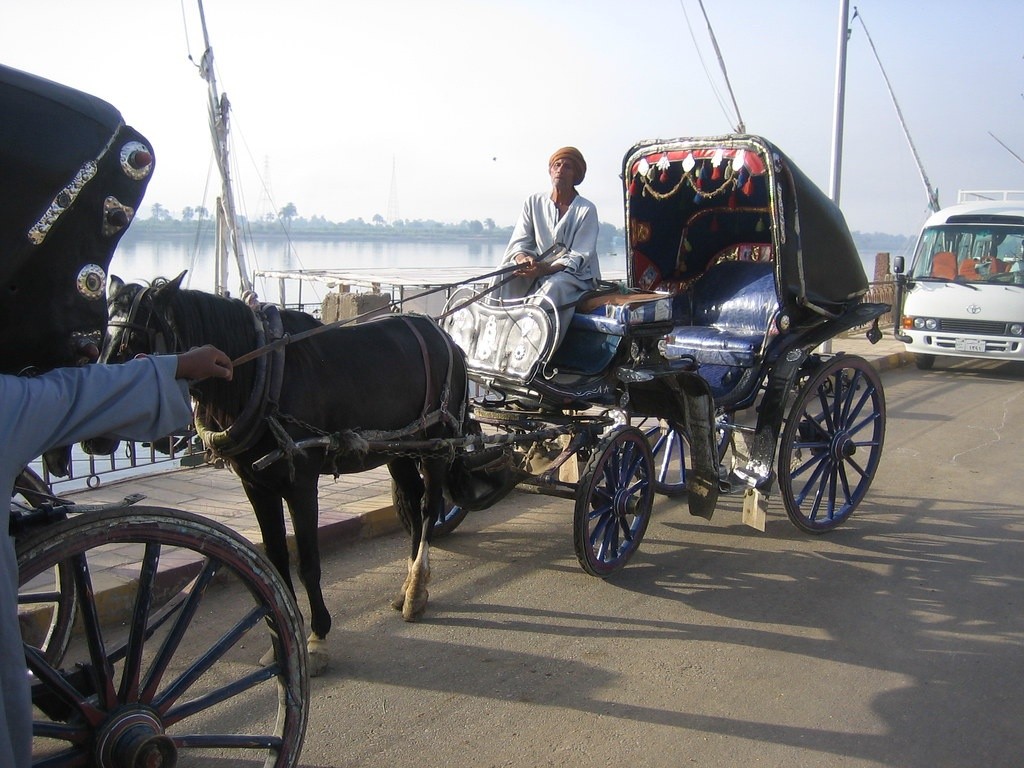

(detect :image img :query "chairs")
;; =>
[991,258,1011,272]
[930,252,958,280]
[658,260,780,407]
[960,258,980,280]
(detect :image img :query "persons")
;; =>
[0,344,234,768]
[1005,240,1024,274]
[487,146,601,378]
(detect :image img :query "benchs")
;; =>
[574,287,673,343]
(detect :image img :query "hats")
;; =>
[550,147,587,186]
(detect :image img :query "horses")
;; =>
[80,270,469,679]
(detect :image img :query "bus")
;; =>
[893,190,1024,370]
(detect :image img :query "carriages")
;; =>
[82,134,892,676]
